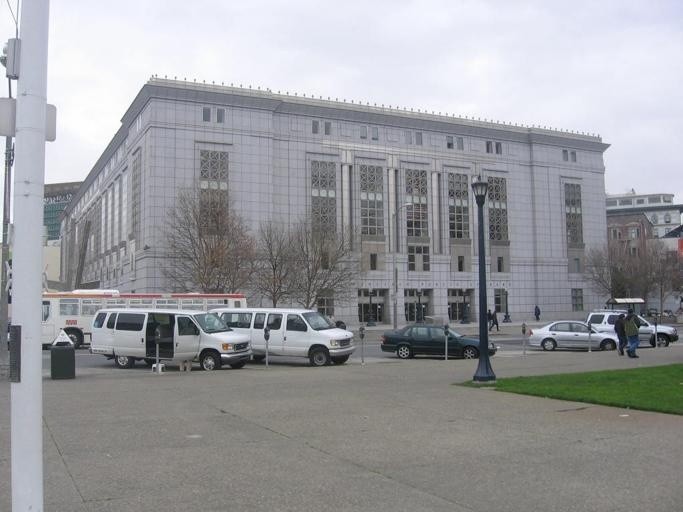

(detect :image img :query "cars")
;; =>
[381,324,497,359]
[527,309,678,351]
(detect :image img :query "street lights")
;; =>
[471,173,497,380]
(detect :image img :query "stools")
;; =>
[151,364,165,373]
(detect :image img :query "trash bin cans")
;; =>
[51,330,75,379]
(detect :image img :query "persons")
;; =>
[615,309,640,358]
[488,310,500,331]
[535,306,540,320]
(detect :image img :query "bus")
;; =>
[42,288,250,350]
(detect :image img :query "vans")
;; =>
[89,307,356,371]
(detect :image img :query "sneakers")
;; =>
[626,349,638,358]
